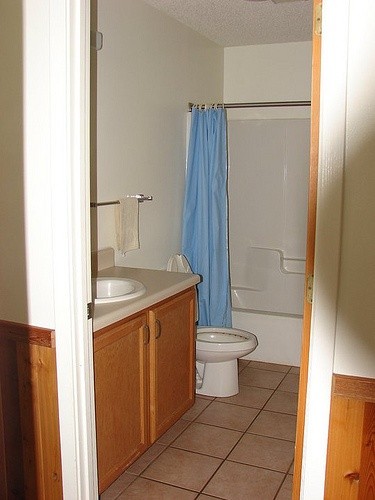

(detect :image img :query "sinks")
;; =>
[91,276,147,305]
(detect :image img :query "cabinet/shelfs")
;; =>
[93,285,196,495]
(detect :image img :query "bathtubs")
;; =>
[231,285,304,368]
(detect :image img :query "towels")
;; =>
[114,196,140,257]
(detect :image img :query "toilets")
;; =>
[165,252,260,399]
